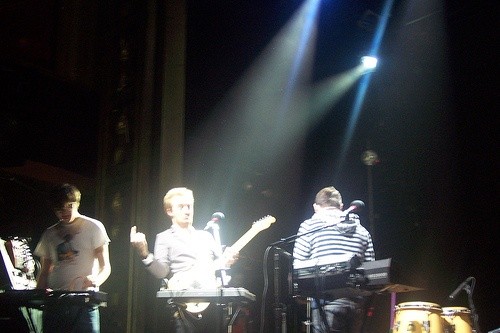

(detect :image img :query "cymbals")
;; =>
[381,282,424,293]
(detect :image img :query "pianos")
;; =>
[154,287,258,303]
[0,286,109,309]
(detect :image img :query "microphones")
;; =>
[204,212,225,232]
[340,200,366,219]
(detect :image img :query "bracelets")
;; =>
[140,252,154,267]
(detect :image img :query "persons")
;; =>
[294,187,376,333]
[33,183,111,333]
[130,188,239,333]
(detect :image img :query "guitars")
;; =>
[215,213,278,271]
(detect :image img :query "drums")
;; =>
[393,300,444,333]
[442,305,474,333]
